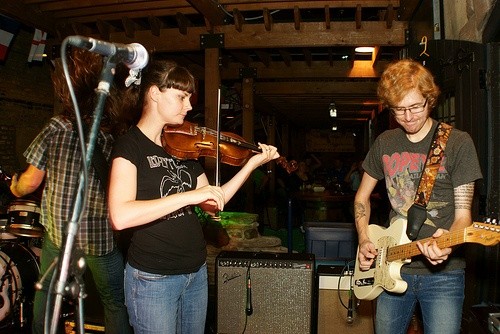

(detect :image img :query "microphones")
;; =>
[347,295,353,323]
[67,35,149,70]
[245,270,254,315]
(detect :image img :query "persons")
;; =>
[107,61,281,334]
[354,58,484,334]
[276,156,393,230]
[10,45,141,334]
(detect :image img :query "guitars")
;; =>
[0,168,42,199]
[352,217,500,300]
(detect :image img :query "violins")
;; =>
[161,120,299,174]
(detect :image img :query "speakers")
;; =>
[215,251,315,333]
[319,274,425,334]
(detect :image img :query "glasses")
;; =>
[389,96,429,116]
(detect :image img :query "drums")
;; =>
[0,219,18,239]
[6,199,44,237]
[0,241,40,329]
